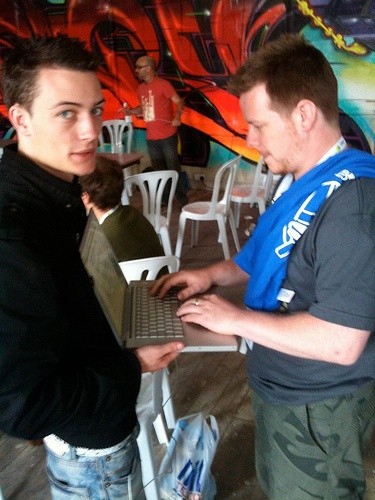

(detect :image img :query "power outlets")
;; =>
[194,173,206,180]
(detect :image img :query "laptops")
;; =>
[78,207,239,351]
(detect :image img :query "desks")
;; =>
[95,152,149,175]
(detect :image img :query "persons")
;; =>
[123,55,189,206]
[77,155,170,281]
[150,34,375,500]
[0,32,184,500]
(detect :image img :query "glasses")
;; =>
[136,65,150,70]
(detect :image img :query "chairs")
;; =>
[134,358,175,500]
[118,256,180,287]
[229,156,275,228]
[118,168,180,258]
[173,155,241,268]
[97,120,133,206]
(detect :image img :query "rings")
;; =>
[196,299,200,306]
